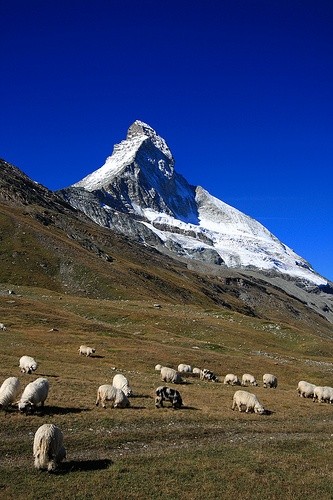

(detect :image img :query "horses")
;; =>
[241,374,258,387]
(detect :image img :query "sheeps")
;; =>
[193,368,203,378]
[79,345,96,357]
[19,356,37,373]
[262,373,277,389]
[296,381,333,405]
[17,377,49,413]
[0,376,21,407]
[113,374,132,397]
[231,390,265,414]
[32,423,67,473]
[178,363,192,372]
[155,386,184,410]
[0,322,7,331]
[201,369,218,382]
[223,374,239,386]
[154,364,179,384]
[96,385,126,409]
